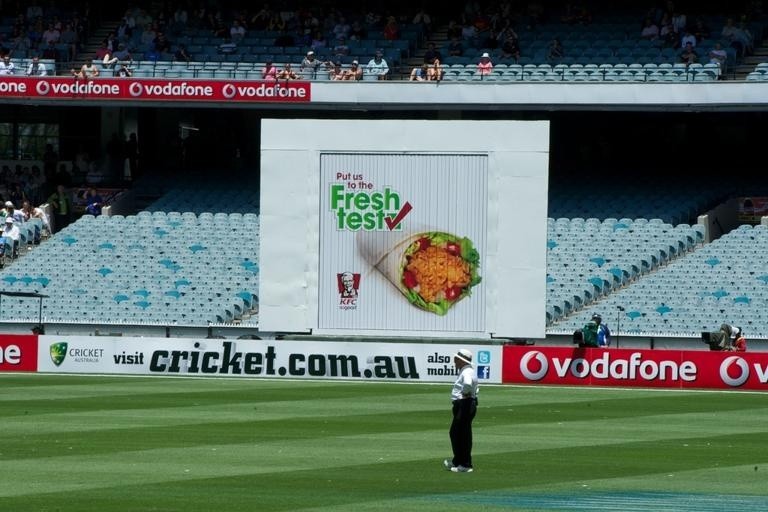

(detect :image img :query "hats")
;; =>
[5,200,15,208]
[454,350,472,365]
[5,217,13,224]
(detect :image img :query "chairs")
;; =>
[0,12,768,82]
[0,178,768,335]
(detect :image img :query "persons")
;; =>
[262,2,432,80]
[1,2,90,59]
[0,132,140,265]
[640,2,752,65]
[525,2,594,57]
[411,2,520,81]
[582,313,611,349]
[727,322,746,354]
[438,346,480,478]
[339,271,360,299]
[194,3,285,45]
[71,1,190,78]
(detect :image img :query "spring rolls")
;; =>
[356,211,483,316]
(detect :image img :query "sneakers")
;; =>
[444,460,473,472]
